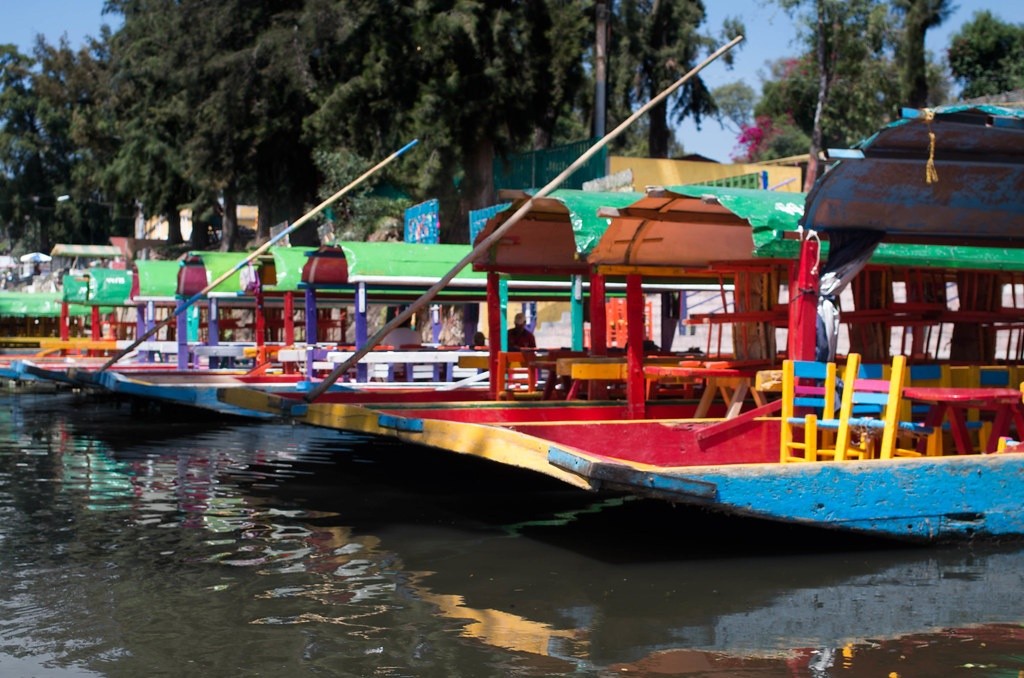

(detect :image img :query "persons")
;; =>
[507,314,542,381]
[470,332,484,348]
[382,317,421,347]
[7,267,14,280]
[34,262,40,272]
[2,273,7,279]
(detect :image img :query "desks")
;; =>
[559,358,687,402]
[901,386,1024,455]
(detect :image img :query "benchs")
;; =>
[529,361,582,400]
[642,365,773,419]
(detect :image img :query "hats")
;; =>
[514,313,526,324]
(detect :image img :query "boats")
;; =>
[0,107,1024,542]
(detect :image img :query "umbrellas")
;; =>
[21,253,52,263]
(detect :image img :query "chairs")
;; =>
[833,352,922,461]
[406,351,439,382]
[446,349,483,382]
[780,360,868,463]
[903,364,952,455]
[367,350,394,382]
[964,363,1018,455]
[305,346,339,376]
[839,363,891,459]
[496,350,544,401]
